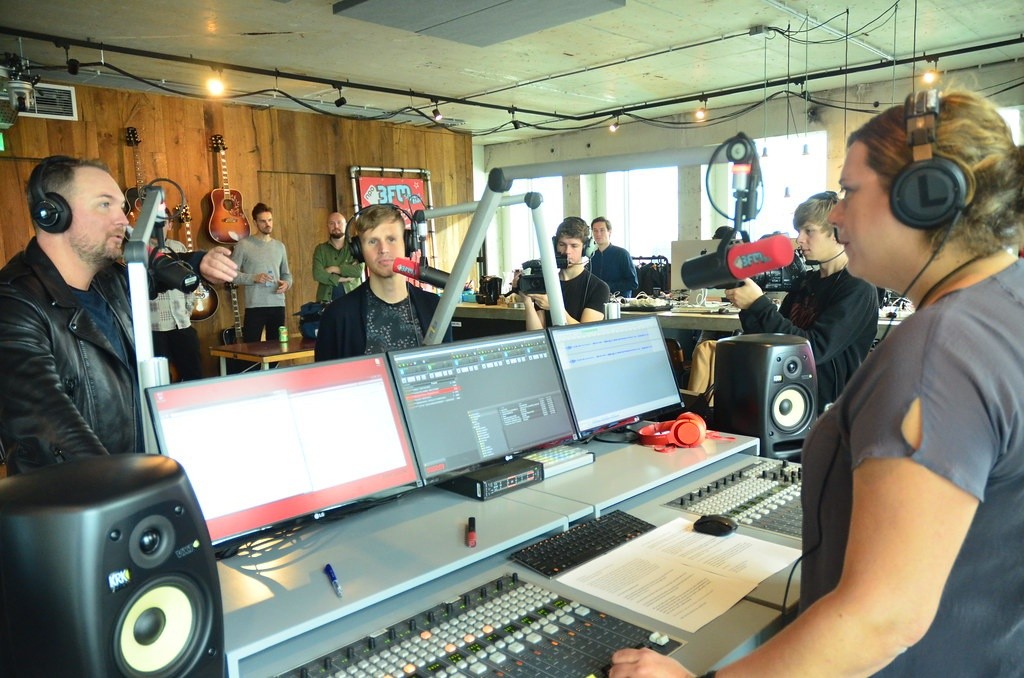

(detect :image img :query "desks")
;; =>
[210,336,317,376]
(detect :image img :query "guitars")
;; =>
[122,127,157,241]
[174,204,220,323]
[205,133,250,246]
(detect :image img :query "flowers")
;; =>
[463,279,473,291]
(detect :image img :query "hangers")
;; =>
[656,255,665,268]
[650,255,656,266]
[636,256,645,267]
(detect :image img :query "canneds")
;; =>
[279,326,289,342]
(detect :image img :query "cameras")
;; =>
[516,251,569,294]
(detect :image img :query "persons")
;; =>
[229,202,294,371]
[585,218,640,301]
[725,189,879,413]
[312,201,453,363]
[617,93,1024,677]
[1,155,238,478]
[700,223,736,257]
[311,210,364,302]
[118,186,206,382]
[512,215,611,331]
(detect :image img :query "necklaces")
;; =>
[912,244,1003,312]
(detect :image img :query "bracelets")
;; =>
[698,671,717,678]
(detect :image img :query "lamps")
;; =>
[697,108,707,118]
[432,109,442,120]
[334,97,346,107]
[68,59,80,75]
[512,120,520,129]
[610,122,620,132]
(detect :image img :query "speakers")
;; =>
[1,453,225,677]
[715,331,819,459]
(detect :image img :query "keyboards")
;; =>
[512,508,656,578]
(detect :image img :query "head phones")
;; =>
[637,411,707,447]
[345,203,419,262]
[888,86,966,228]
[550,216,598,259]
[813,191,843,245]
[30,156,81,232]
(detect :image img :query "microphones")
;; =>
[145,243,199,300]
[393,257,451,288]
[680,235,795,290]
[805,249,846,266]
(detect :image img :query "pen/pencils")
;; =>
[323,563,346,599]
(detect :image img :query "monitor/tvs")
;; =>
[144,312,685,548]
[671,238,730,297]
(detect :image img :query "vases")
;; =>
[463,290,474,302]
